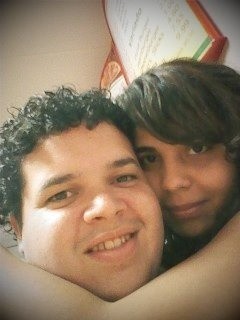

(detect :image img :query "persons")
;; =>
[0,87,168,303]
[0,58,240,320]
